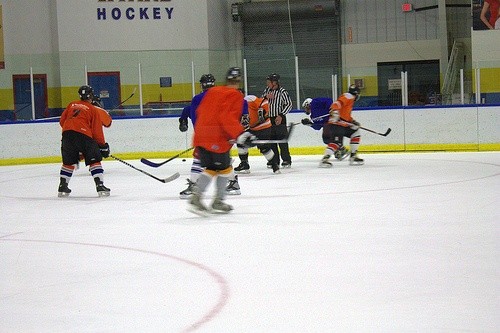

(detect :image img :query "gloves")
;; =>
[240,115,250,131]
[99,142,110,158]
[329,111,339,123]
[352,120,360,127]
[246,136,261,147]
[301,119,314,126]
[179,117,188,132]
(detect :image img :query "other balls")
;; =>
[182,158,186,161]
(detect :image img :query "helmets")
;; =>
[78,84,93,94]
[302,97,313,108]
[91,95,100,104]
[199,74,215,82]
[225,67,241,80]
[265,73,280,81]
[349,84,360,94]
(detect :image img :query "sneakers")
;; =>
[187,195,207,217]
[350,157,364,166]
[272,163,280,174]
[210,198,232,214]
[267,161,280,168]
[334,147,348,160]
[281,161,291,169]
[94,177,110,197]
[233,161,251,174]
[57,177,72,197]
[225,174,241,194]
[318,155,332,167]
[180,179,198,198]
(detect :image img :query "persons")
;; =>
[58,85,110,192]
[234,88,280,173]
[189,68,255,210]
[320,85,364,166]
[77,95,112,171]
[301,98,352,158]
[179,74,249,196]
[480,0,500,30]
[259,74,292,166]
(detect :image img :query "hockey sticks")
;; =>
[106,152,181,184]
[337,123,393,137]
[107,87,137,115]
[140,147,196,169]
[286,114,331,127]
[227,121,293,144]
